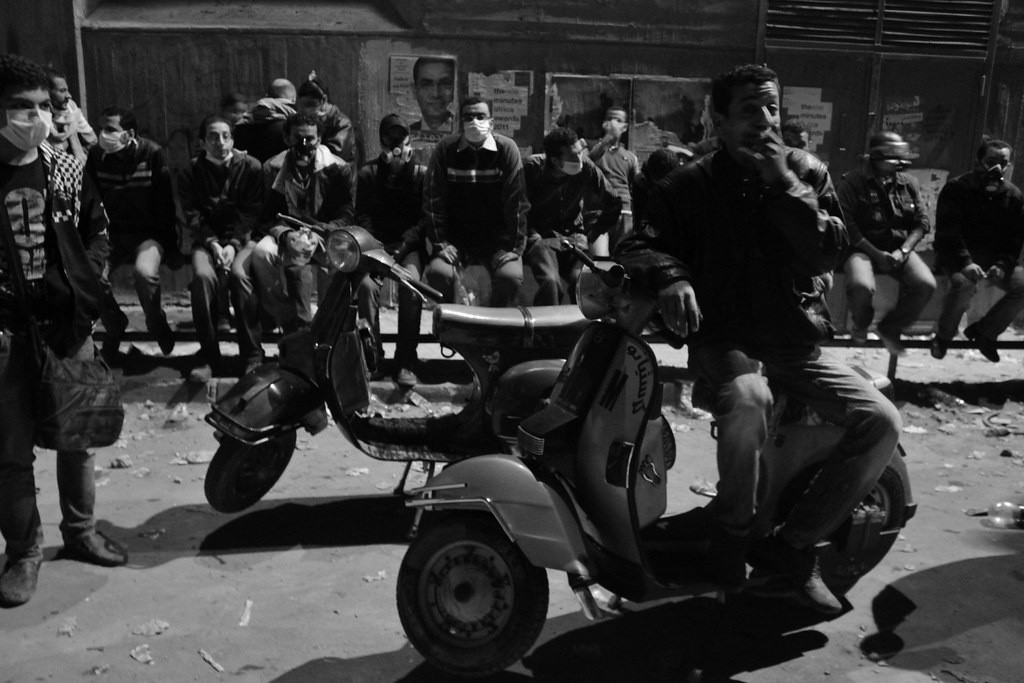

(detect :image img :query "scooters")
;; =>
[203,202,599,514]
[396,210,916,681]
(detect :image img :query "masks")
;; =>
[98,129,130,154]
[463,119,493,143]
[300,103,329,119]
[559,158,584,175]
[287,140,320,168]
[0,108,55,150]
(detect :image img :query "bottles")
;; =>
[289,227,318,267]
[987,501,1024,529]
[926,388,966,407]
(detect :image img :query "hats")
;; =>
[378,113,412,137]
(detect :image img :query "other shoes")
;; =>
[701,503,748,596]
[872,323,906,356]
[188,352,224,385]
[764,535,845,615]
[240,357,264,380]
[396,364,418,386]
[850,319,871,345]
[102,315,129,356]
[151,321,177,354]
[370,364,390,381]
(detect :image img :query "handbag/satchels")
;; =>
[29,337,126,450]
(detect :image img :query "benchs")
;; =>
[87,248,1024,352]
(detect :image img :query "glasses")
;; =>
[461,113,489,121]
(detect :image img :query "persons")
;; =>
[424,98,530,307]
[521,109,682,306]
[177,81,431,388]
[410,57,455,133]
[43,68,184,363]
[838,133,937,354]
[931,140,1024,362]
[784,118,811,151]
[619,66,901,614]
[0,55,129,607]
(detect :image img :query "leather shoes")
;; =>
[63,530,127,567]
[0,552,44,608]
[963,320,1001,363]
[930,332,950,359]
